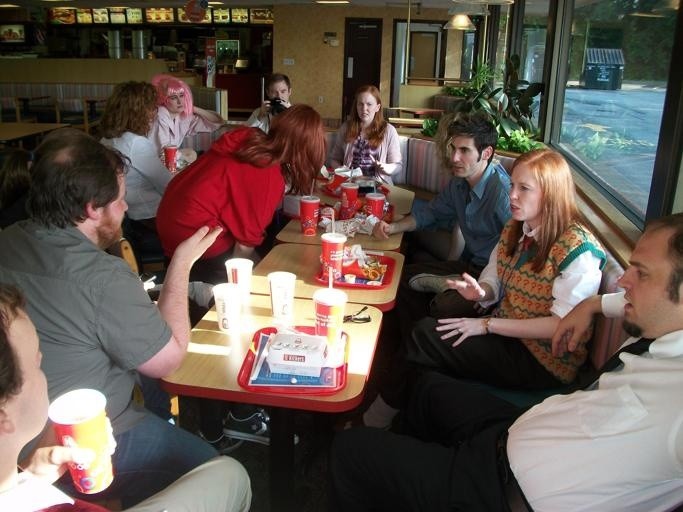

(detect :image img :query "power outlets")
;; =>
[282,58,290,64]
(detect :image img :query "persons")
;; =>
[0,144,224,499]
[244,74,293,135]
[95,81,183,255]
[0,282,251,512]
[329,85,402,186]
[328,212,683,512]
[147,74,225,164]
[330,149,608,435]
[154,102,328,280]
[372,116,516,321]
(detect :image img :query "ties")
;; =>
[576,338,657,391]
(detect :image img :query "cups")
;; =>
[300,197,321,237]
[365,193,385,219]
[225,258,254,305]
[47,389,115,494]
[164,145,179,172]
[335,167,351,188]
[321,233,346,279]
[341,183,358,218]
[314,288,347,340]
[212,283,245,333]
[268,271,296,317]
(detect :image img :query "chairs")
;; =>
[113,235,167,291]
[120,218,170,276]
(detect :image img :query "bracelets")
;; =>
[481,315,492,336]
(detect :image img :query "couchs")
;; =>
[407,135,523,202]
[478,212,633,408]
[323,127,410,191]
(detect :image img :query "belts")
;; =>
[495,429,531,512]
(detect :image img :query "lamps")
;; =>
[441,14,478,32]
[315,0,352,4]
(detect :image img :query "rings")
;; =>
[456,328,462,334]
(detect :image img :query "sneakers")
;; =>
[222,411,300,445]
[409,272,466,294]
[191,431,243,455]
[331,411,393,436]
